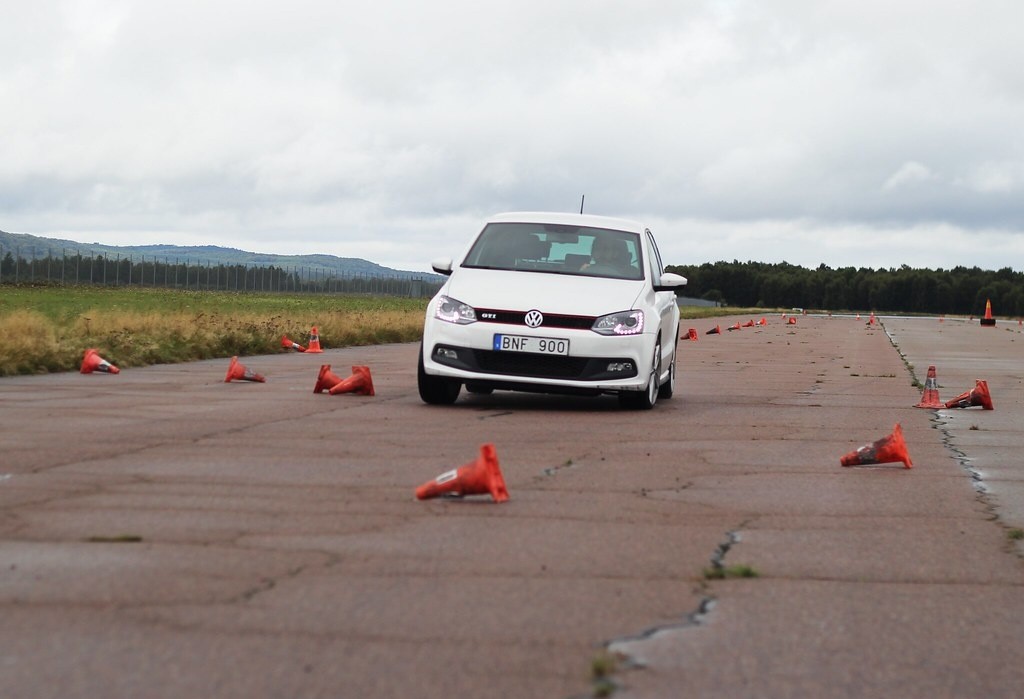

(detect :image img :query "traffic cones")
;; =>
[1018,319,1023,329]
[789,318,797,324]
[781,312,786,319]
[224,355,266,382]
[80,349,120,374]
[416,443,511,504]
[680,329,698,340]
[855,313,861,321]
[755,318,766,325]
[314,365,343,393]
[840,422,913,469]
[912,366,946,409]
[329,366,374,396]
[726,322,741,330]
[984,299,993,319]
[305,327,323,353]
[939,318,944,324]
[706,325,720,334]
[742,319,754,327]
[946,380,994,410]
[283,335,306,352]
[870,312,875,325]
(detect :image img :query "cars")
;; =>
[417,196,688,408]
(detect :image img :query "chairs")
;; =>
[511,235,542,269]
[613,240,631,274]
